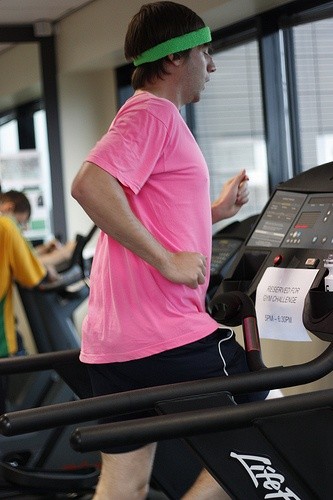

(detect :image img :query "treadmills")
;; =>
[0,161,333,500]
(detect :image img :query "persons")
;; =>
[70,0,269,500]
[1,190,76,414]
